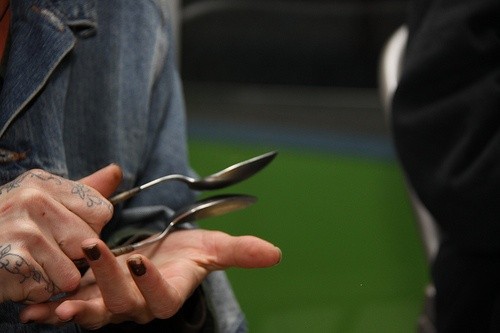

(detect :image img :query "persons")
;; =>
[391,0,500,333]
[0,0,282,333]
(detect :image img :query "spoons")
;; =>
[111,152,277,206]
[111,194,257,256]
